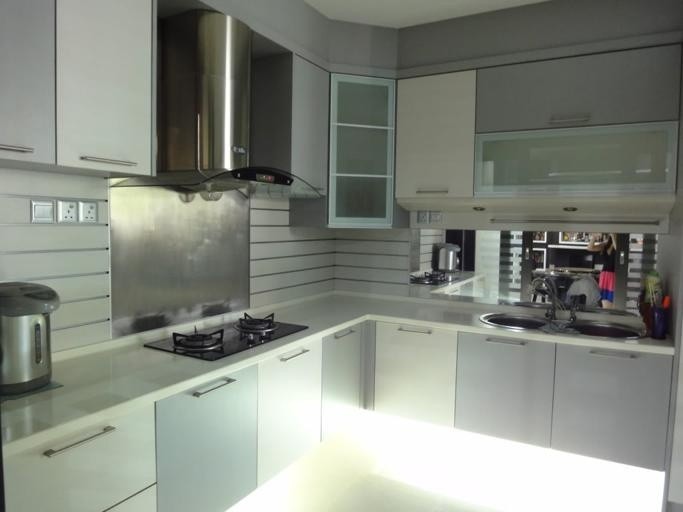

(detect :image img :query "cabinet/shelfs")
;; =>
[394,60,478,214]
[155,356,257,512]
[472,118,682,217]
[322,312,377,445]
[454,323,554,452]
[438,275,488,298]
[376,310,457,428]
[286,48,332,201]
[55,0,157,179]
[256,330,322,512]
[285,58,396,233]
[553,333,682,473]
[0,395,156,512]
[472,27,683,135]
[0,0,55,179]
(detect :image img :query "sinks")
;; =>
[565,318,647,340]
[478,311,549,331]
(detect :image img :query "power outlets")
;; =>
[55,200,78,225]
[77,200,99,225]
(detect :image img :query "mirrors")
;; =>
[406,226,660,319]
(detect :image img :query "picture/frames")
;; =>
[557,231,603,246]
[530,246,546,270]
[529,230,546,245]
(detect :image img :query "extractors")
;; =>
[110,11,325,203]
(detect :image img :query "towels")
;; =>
[561,275,602,307]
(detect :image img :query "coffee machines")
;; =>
[0,278,60,394]
[435,241,460,272]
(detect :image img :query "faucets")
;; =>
[530,276,579,328]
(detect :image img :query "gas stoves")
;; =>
[143,308,310,360]
[410,269,456,286]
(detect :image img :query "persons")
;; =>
[585,230,618,308]
